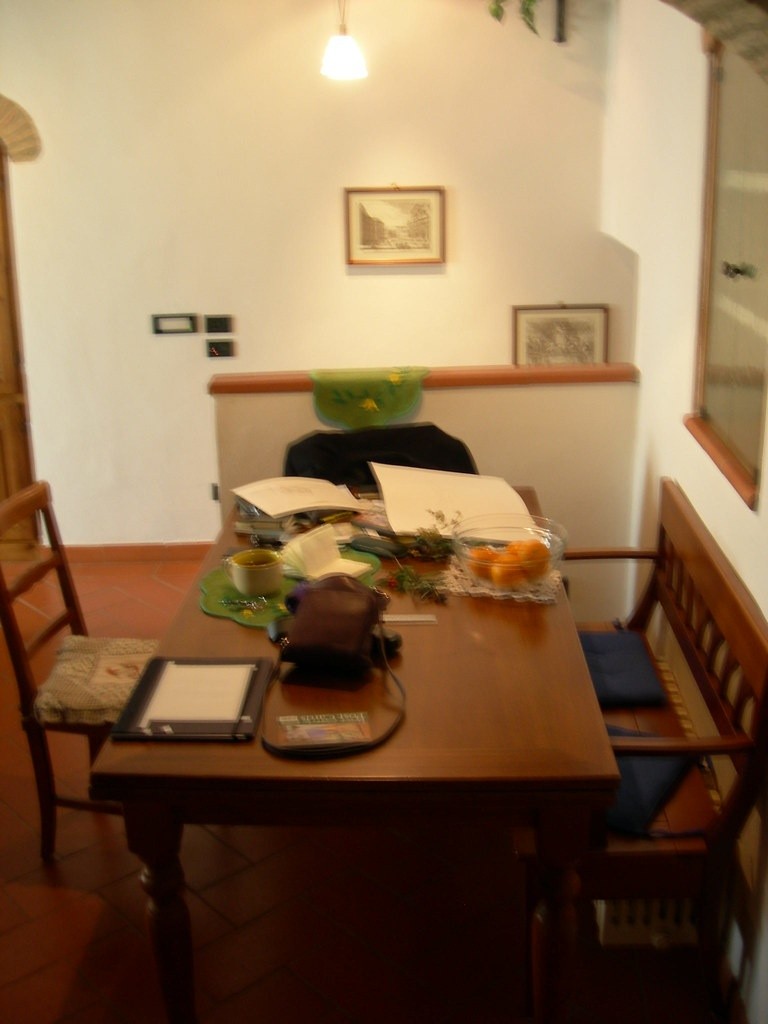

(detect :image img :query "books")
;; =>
[230,477,396,549]
[282,523,372,582]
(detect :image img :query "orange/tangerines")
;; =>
[467,539,552,591]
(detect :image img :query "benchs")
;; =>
[512,477,767,1002]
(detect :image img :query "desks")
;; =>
[86,488,624,1024]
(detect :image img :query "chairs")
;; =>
[0,484,159,865]
[283,422,479,492]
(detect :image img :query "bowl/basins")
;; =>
[451,514,569,590]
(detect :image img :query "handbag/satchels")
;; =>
[279,571,391,660]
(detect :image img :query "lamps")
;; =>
[319,0,369,82]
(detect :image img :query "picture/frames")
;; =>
[512,304,609,365]
[344,187,447,265]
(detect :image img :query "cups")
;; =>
[225,549,282,597]
